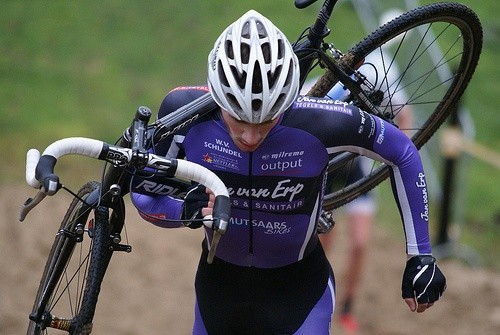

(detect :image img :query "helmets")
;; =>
[206,9,300,124]
[357,51,399,94]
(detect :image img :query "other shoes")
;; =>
[339,311,358,333]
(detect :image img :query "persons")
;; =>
[130,9,448,335]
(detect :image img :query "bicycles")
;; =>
[18,0,483,335]
